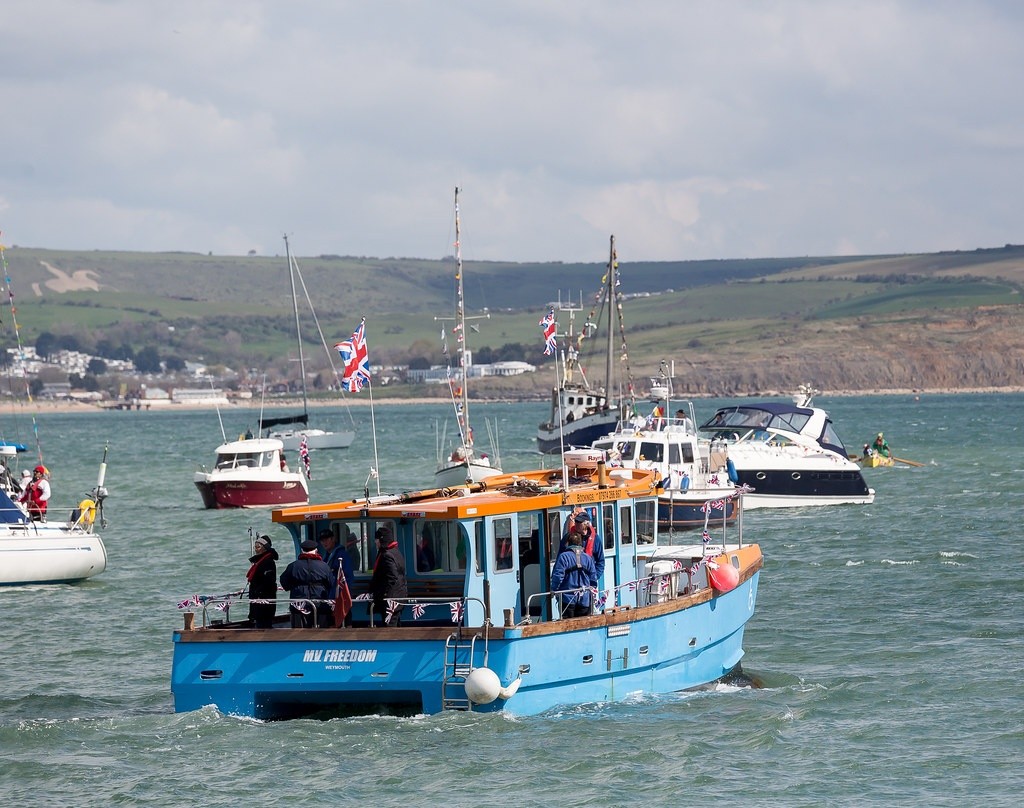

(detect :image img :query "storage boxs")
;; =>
[645,560,679,604]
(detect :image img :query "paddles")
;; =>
[893,458,924,467]
[848,454,857,458]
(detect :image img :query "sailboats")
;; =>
[534,234,647,456]
[250,229,357,452]
[0,230,112,587]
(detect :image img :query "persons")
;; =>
[676,409,688,425]
[320,528,353,627]
[714,414,726,437]
[280,540,334,629]
[369,525,407,629]
[279,450,286,471]
[862,444,872,456]
[19,471,33,489]
[332,524,361,570]
[873,432,894,459]
[551,512,605,581]
[17,466,52,521]
[246,535,279,630]
[550,533,597,619]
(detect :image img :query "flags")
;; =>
[672,470,752,544]
[333,320,370,394]
[564,255,637,416]
[573,552,722,609]
[537,308,558,354]
[176,586,465,622]
[335,567,352,626]
[299,436,311,481]
[651,407,665,427]
[441,200,488,461]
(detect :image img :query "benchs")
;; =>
[349,576,464,626]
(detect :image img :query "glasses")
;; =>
[33,470,40,472]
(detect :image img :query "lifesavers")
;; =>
[79,499,96,522]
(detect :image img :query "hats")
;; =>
[573,512,590,522]
[376,527,393,548]
[301,540,318,552]
[678,410,685,414]
[35,466,44,474]
[318,529,334,539]
[256,535,272,548]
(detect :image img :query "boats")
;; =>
[169,184,764,722]
[698,379,876,510]
[585,354,757,534]
[189,374,312,511]
[860,450,895,469]
